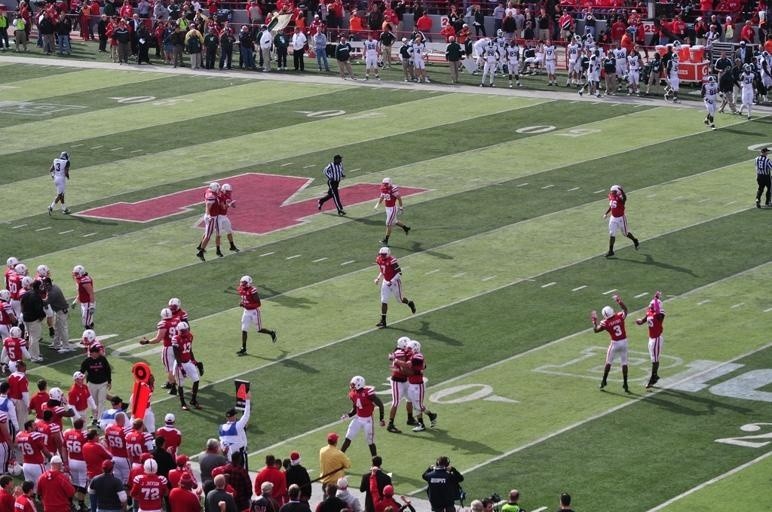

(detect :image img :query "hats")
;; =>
[333,154,343,160]
[382,485,393,496]
[225,407,239,418]
[175,454,188,464]
[260,481,273,492]
[206,438,219,449]
[72,371,85,382]
[164,413,175,423]
[335,478,348,489]
[49,455,62,464]
[110,395,122,405]
[140,453,153,462]
[89,343,104,352]
[177,471,194,487]
[327,432,339,441]
[101,459,114,470]
[760,148,770,153]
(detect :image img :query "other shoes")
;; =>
[352,77,357,80]
[342,76,346,80]
[451,79,457,84]
[516,82,522,87]
[259,66,304,72]
[376,76,381,80]
[547,83,552,86]
[480,84,485,87]
[365,76,368,81]
[489,83,494,88]
[663,87,681,104]
[508,82,513,88]
[554,83,559,87]
[325,69,330,72]
[320,68,322,72]
[566,78,659,98]
[404,75,431,83]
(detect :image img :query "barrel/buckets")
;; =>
[655,44,704,63]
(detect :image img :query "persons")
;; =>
[315,154,349,219]
[556,491,574,512]
[47,150,74,216]
[590,294,634,395]
[374,177,411,248]
[374,246,417,329]
[195,180,224,262]
[601,183,639,259]
[196,183,241,254]
[753,147,771,209]
[630,289,666,390]
[699,76,720,132]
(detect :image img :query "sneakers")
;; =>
[386,424,401,434]
[196,243,241,262]
[430,412,437,427]
[755,198,771,209]
[379,237,387,246]
[703,118,716,131]
[406,417,425,431]
[160,381,201,410]
[376,320,386,327]
[316,198,345,216]
[236,347,246,356]
[600,374,660,392]
[23,326,73,362]
[408,300,416,314]
[270,329,276,343]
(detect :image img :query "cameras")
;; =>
[492,493,502,504]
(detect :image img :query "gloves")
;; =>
[1,365,7,373]
[374,279,379,285]
[87,305,96,313]
[399,206,403,215]
[654,290,662,297]
[70,299,76,307]
[340,413,349,421]
[177,365,187,376]
[140,336,149,346]
[591,309,597,324]
[379,418,384,426]
[612,294,621,305]
[635,318,641,324]
[385,280,391,287]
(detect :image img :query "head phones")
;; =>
[436,456,450,467]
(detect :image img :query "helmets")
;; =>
[6,256,50,289]
[707,76,716,82]
[382,177,392,188]
[240,274,253,288]
[671,53,678,59]
[143,458,158,474]
[349,375,365,390]
[742,50,769,72]
[159,297,189,333]
[649,299,662,311]
[71,265,85,276]
[395,336,421,356]
[488,29,505,48]
[0,290,11,300]
[609,185,622,197]
[209,182,232,194]
[601,304,616,320]
[82,329,95,345]
[7,461,24,477]
[60,151,69,160]
[9,325,22,338]
[378,246,392,258]
[48,386,63,401]
